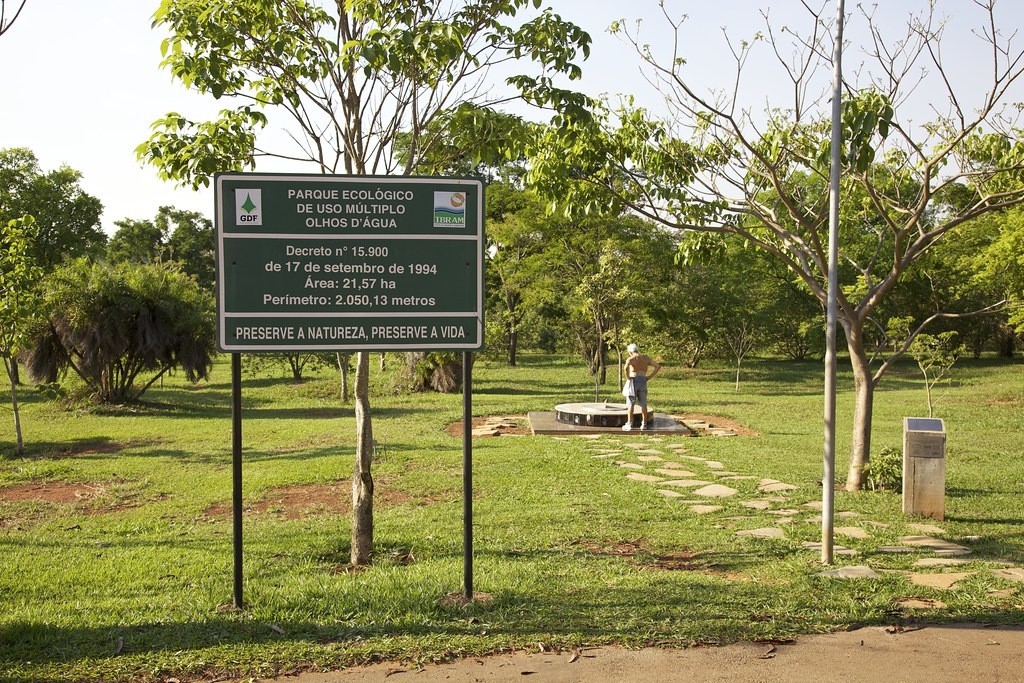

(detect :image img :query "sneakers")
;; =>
[621,423,632,430]
[639,424,648,430]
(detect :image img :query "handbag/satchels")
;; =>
[622,378,635,397]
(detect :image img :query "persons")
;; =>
[621,342,661,430]
